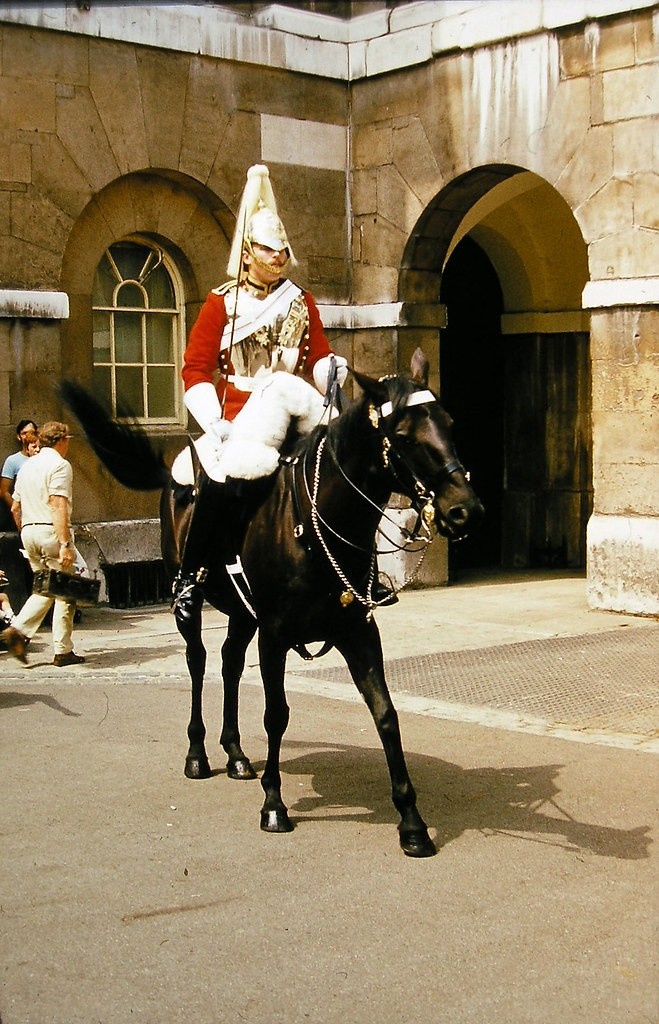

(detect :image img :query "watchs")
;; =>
[60,540,71,548]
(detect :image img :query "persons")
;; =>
[173,162,344,631]
[0,593,16,632]
[22,431,41,459]
[1,421,89,667]
[3,421,34,509]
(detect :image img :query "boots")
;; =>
[368,565,400,606]
[171,436,227,626]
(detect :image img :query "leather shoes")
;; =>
[53,650,86,668]
[1,626,29,665]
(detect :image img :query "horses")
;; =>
[48,346,484,859]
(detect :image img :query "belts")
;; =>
[219,373,264,392]
[21,523,73,530]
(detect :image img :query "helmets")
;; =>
[241,163,290,251]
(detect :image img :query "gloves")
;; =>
[184,381,232,441]
[313,352,347,396]
